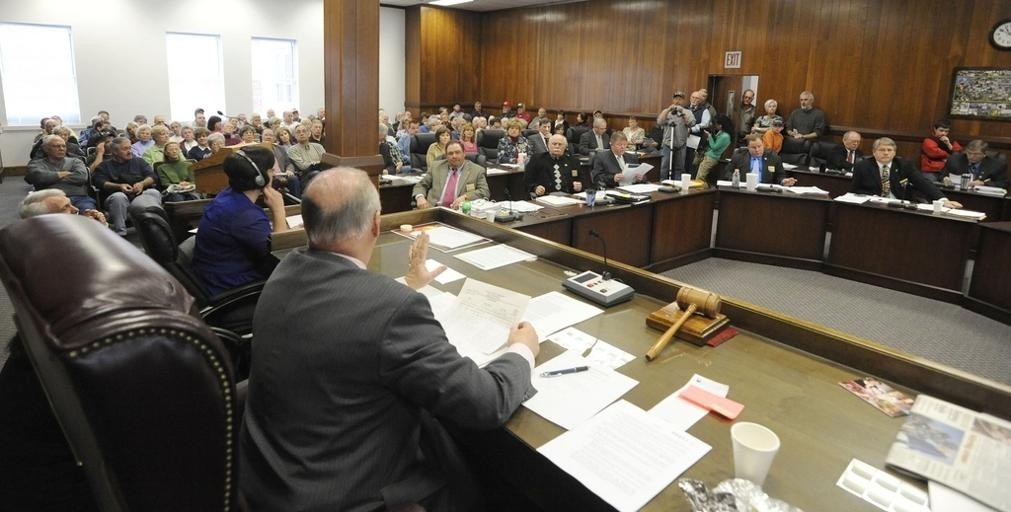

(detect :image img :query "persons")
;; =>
[24,104,329,236]
[234,165,539,511]
[192,145,287,333]
[380,87,1011,210]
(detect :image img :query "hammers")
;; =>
[646,286,721,361]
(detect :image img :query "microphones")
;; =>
[504,187,513,214]
[588,228,612,280]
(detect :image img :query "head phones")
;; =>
[234,150,270,188]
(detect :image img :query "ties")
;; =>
[881,164,890,195]
[544,138,548,144]
[970,163,976,173]
[553,157,562,190]
[751,158,760,175]
[442,168,458,207]
[848,151,853,163]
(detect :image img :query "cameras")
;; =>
[671,104,683,118]
[700,125,715,134]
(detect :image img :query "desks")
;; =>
[578,151,663,184]
[718,159,802,178]
[486,200,572,249]
[380,163,527,213]
[529,185,653,275]
[792,165,853,197]
[616,179,718,272]
[821,193,981,304]
[963,220,1010,322]
[268,208,1010,512]
[716,183,829,272]
[933,183,1010,222]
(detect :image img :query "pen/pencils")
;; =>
[540,365,590,378]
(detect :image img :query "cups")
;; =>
[586,189,596,206]
[960,174,970,191]
[932,200,944,216]
[485,210,496,222]
[745,173,757,191]
[518,153,526,166]
[729,422,781,488]
[681,173,691,191]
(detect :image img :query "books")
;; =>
[947,210,988,221]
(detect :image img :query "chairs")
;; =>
[808,142,839,169]
[476,128,504,162]
[780,135,807,163]
[129,196,266,313]
[566,127,591,152]
[521,130,538,136]
[1,214,235,512]
[409,134,440,170]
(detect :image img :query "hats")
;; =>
[673,92,685,98]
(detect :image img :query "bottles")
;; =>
[733,169,740,187]
[463,197,473,216]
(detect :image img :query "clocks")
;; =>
[988,20,1007,49]
